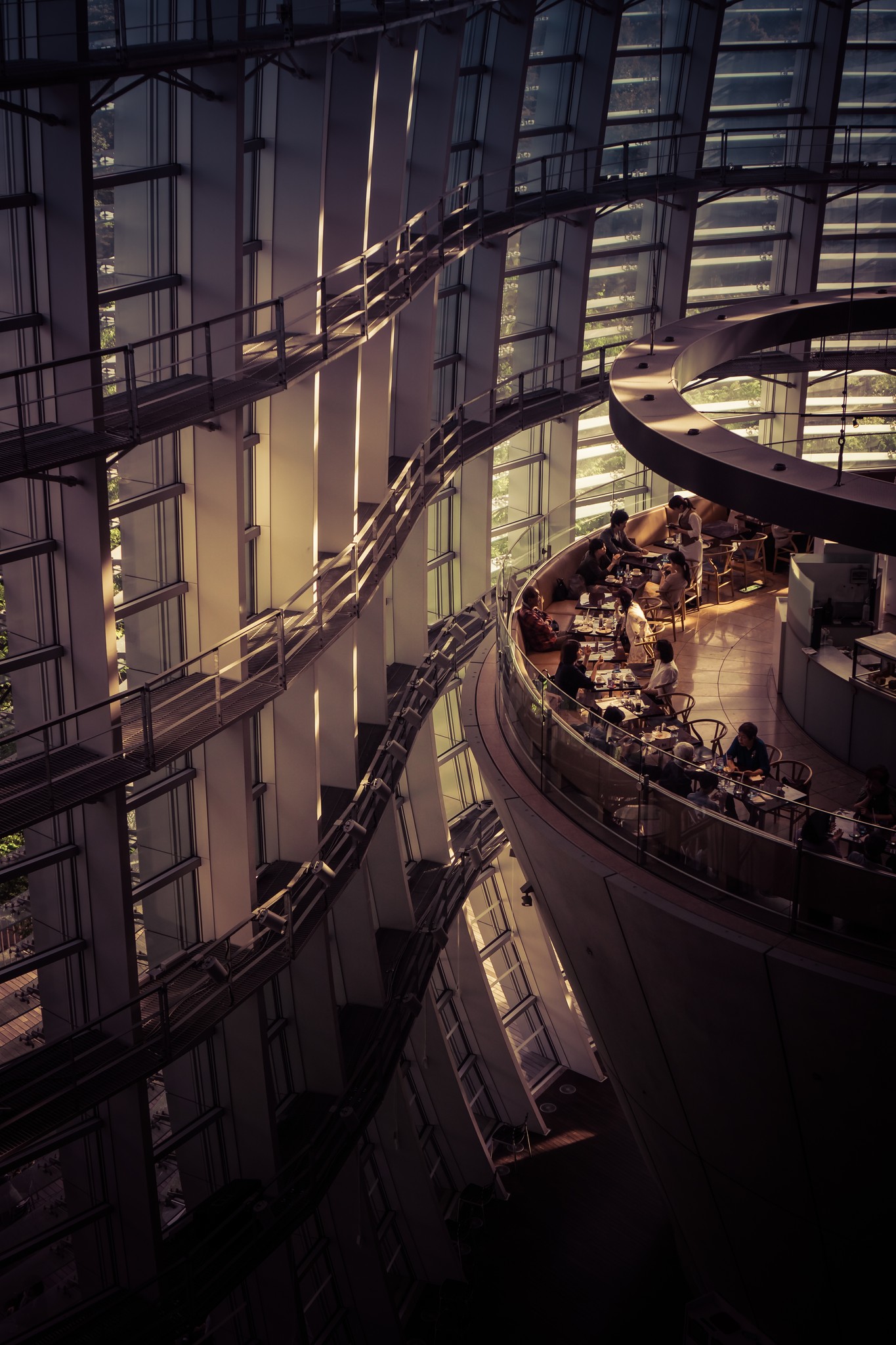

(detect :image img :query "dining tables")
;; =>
[568,614,619,642]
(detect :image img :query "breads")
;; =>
[598,654,603,662]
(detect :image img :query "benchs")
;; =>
[512,495,847,951]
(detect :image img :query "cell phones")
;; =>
[617,553,625,560]
[625,738,635,745]
[665,525,677,530]
[657,563,665,573]
[628,683,641,687]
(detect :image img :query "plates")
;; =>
[651,725,679,739]
[641,737,655,742]
[594,673,635,688]
[605,571,643,582]
[577,618,616,633]
[661,541,676,562]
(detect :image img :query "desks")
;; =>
[826,808,896,857]
[594,568,653,590]
[686,756,808,832]
[631,722,701,768]
[616,694,666,731]
[584,668,642,697]
[701,520,751,563]
[735,513,772,533]
[653,534,713,551]
[575,591,623,617]
[577,640,628,668]
[620,550,665,582]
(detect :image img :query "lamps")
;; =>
[392,993,421,1012]
[252,1196,276,1227]
[203,599,490,988]
[341,1104,360,1131]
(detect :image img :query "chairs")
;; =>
[768,758,813,842]
[667,558,701,619]
[682,720,727,792]
[727,532,768,588]
[638,596,662,640]
[772,530,816,574]
[765,742,783,767]
[640,669,676,714]
[445,1110,532,1255]
[644,693,695,736]
[702,543,736,602]
[645,586,686,642]
[621,640,657,678]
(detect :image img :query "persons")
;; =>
[522,496,896,872]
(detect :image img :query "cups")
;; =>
[726,755,732,771]
[760,784,764,791]
[712,758,717,767]
[670,731,678,739]
[606,566,640,581]
[622,692,630,700]
[624,699,641,714]
[736,776,743,793]
[853,818,861,837]
[750,785,759,797]
[666,537,674,543]
[596,663,634,687]
[662,553,669,560]
[643,733,652,740]
[717,757,725,769]
[655,723,666,731]
[583,612,614,627]
[676,533,680,542]
[705,761,712,770]
